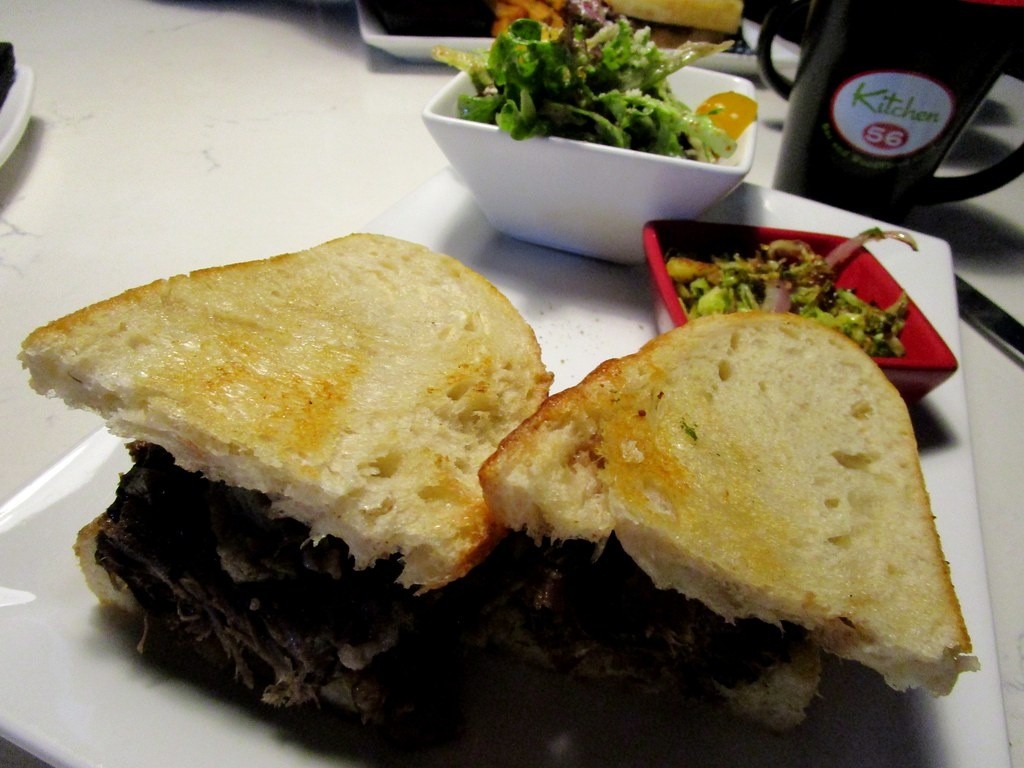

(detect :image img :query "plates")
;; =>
[1,65,39,167]
[1,166,1010,768]
[358,1,800,75]
[643,218,959,406]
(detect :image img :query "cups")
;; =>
[772,0,1023,224]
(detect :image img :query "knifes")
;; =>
[955,273,1024,355]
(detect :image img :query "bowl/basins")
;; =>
[421,65,759,262]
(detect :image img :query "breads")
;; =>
[15,232,982,735]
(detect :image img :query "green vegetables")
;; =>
[431,6,738,163]
[663,238,914,364]
